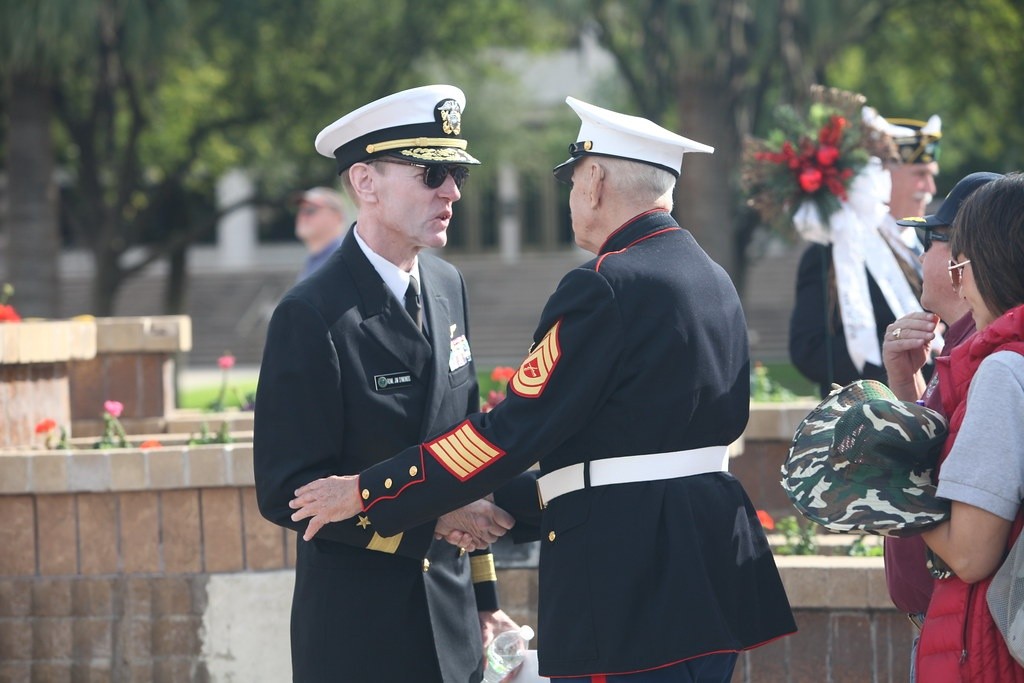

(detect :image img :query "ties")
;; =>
[405,276,432,347]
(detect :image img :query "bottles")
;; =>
[481,624,535,683]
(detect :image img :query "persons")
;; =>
[779,117,1024,683]
[253,84,524,683]
[289,95,799,683]
[294,186,346,283]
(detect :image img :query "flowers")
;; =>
[738,86,870,232]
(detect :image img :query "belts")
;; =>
[535,446,730,508]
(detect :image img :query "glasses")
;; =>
[298,207,327,215]
[365,158,470,189]
[948,259,972,294]
[924,226,950,253]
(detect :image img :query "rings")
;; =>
[892,328,901,337]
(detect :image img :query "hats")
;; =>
[291,187,347,214]
[782,378,950,536]
[882,118,943,164]
[896,172,1004,227]
[553,96,715,184]
[314,84,482,176]
